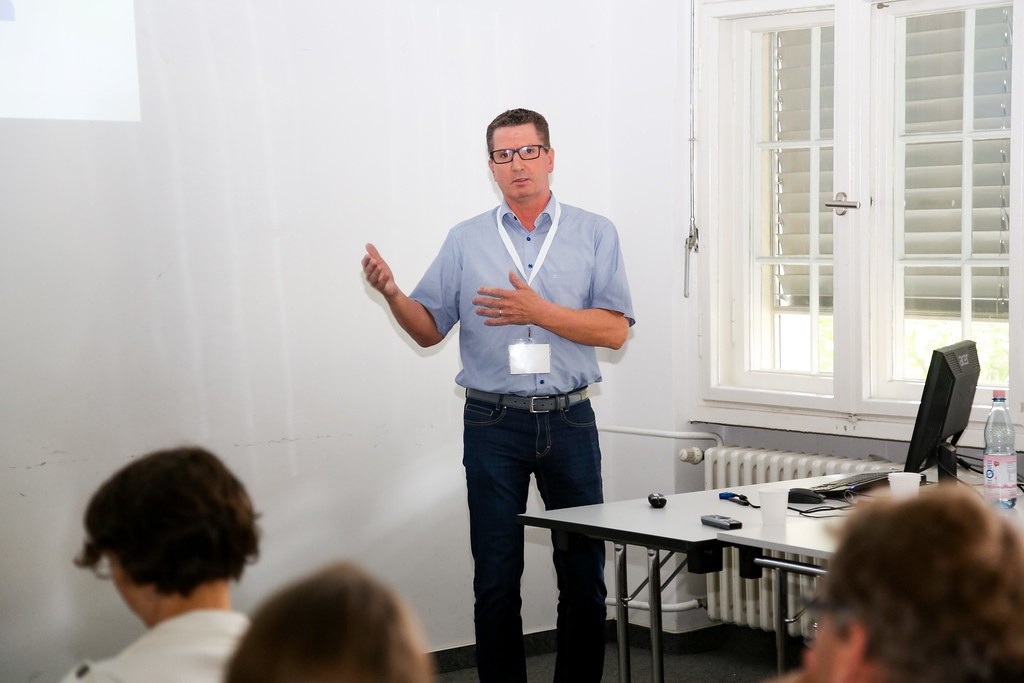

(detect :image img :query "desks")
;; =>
[517,472,924,683]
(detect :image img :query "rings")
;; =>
[499,307,501,316]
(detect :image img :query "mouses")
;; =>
[788,488,826,504]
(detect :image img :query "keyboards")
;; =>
[810,470,895,500]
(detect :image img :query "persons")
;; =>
[60,447,264,683]
[361,108,638,683]
[223,562,432,683]
[780,485,1022,683]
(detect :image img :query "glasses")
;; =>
[489,145,549,165]
[93,556,129,581]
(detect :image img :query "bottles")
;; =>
[982,389,1018,510]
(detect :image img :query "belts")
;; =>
[466,385,589,414]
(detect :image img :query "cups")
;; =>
[887,472,921,496]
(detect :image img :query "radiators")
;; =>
[703,445,905,640]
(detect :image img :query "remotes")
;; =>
[648,493,667,508]
[701,515,742,530]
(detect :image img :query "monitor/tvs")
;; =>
[904,339,981,485]
